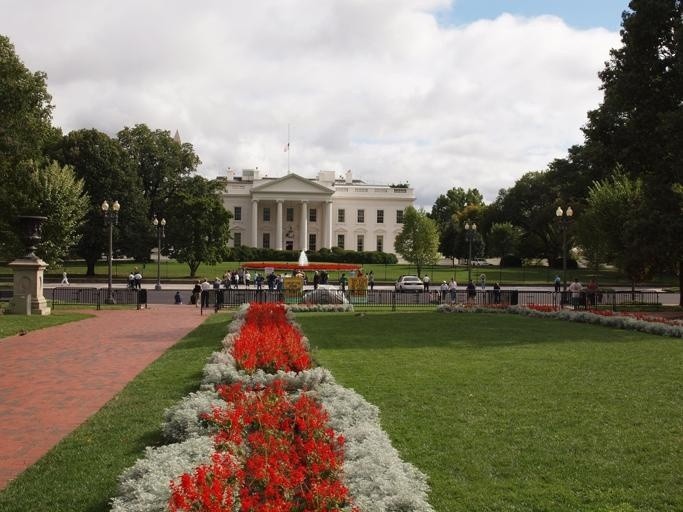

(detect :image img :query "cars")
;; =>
[469,257,489,267]
[393,275,424,292]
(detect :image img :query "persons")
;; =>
[422,274,431,292]
[356,269,363,277]
[588,277,596,307]
[492,282,500,304]
[60,271,69,286]
[128,271,134,289]
[448,277,457,304]
[192,278,200,307]
[134,271,141,289]
[339,273,349,291]
[199,278,210,308]
[466,278,476,304]
[553,273,562,294]
[592,277,603,304]
[568,277,583,309]
[185,268,329,294]
[438,278,448,299]
[479,273,486,290]
[367,268,376,288]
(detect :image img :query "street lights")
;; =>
[462,218,476,289]
[555,206,573,290]
[151,218,167,290]
[97,198,120,308]
